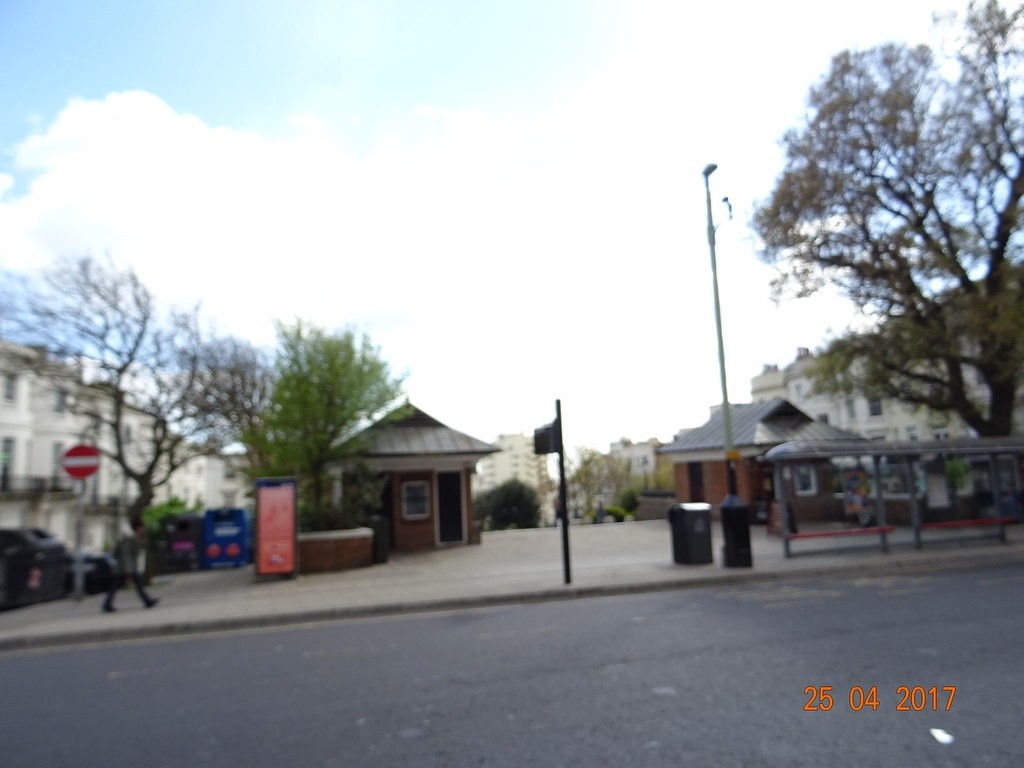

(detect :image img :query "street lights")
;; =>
[701,162,754,568]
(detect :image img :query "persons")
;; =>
[102,517,160,612]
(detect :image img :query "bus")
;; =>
[160,508,251,571]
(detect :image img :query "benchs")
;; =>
[919,518,1010,529]
[788,528,896,540]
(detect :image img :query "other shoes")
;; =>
[104,605,117,612]
[146,599,158,607]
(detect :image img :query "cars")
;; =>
[1,527,74,609]
[69,549,123,594]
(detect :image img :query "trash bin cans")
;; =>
[159,515,203,571]
[665,502,713,564]
[0,526,67,608]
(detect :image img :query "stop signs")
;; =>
[62,445,100,478]
